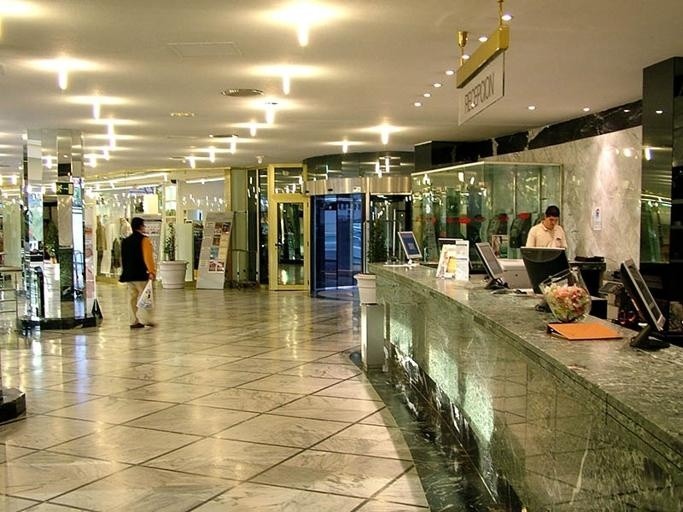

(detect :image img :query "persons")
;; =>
[526,205,569,250]
[118,217,157,328]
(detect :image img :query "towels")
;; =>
[361,303,385,369]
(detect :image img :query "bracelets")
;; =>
[540,266,594,327]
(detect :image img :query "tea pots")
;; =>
[21,199,95,322]
[187,179,224,269]
[274,167,303,193]
[277,202,304,285]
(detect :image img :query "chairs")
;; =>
[130,322,158,329]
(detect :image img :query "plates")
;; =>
[133,214,176,280]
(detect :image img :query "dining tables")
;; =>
[353,202,387,304]
[42,220,61,318]
[157,222,189,288]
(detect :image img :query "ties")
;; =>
[475,242,506,289]
[620,257,670,350]
[398,231,423,267]
[438,238,473,278]
[520,246,571,313]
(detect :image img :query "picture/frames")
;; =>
[0,266,28,336]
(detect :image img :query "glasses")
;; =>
[95,298,103,319]
